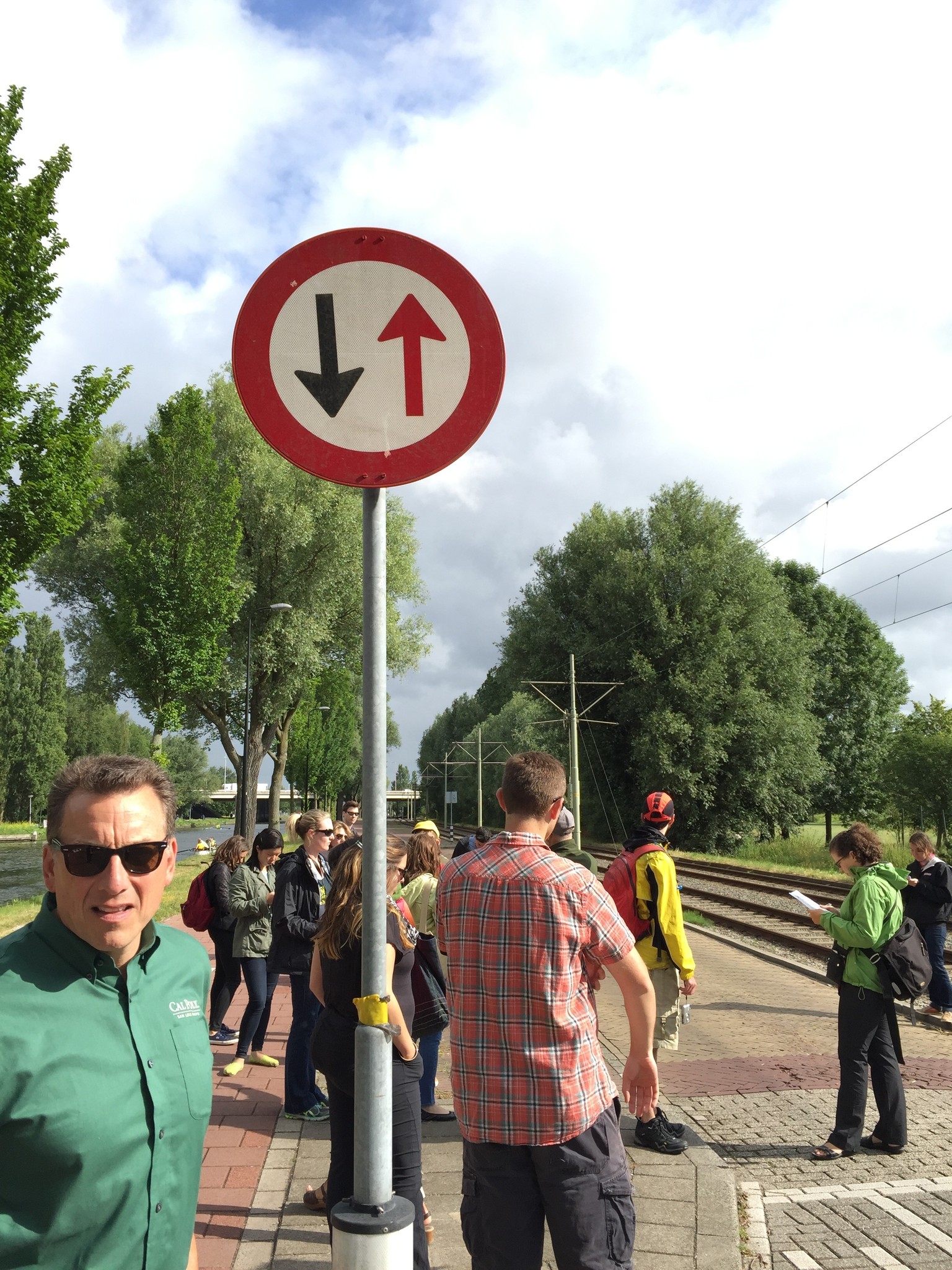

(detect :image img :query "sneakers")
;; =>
[634,1115,688,1152]
[915,1004,952,1022]
[654,1106,684,1137]
[284,1095,330,1121]
[208,1023,240,1044]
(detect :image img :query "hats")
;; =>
[642,792,673,822]
[411,820,440,838]
[553,806,575,836]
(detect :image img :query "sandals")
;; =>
[303,1179,330,1211]
[811,1132,908,1160]
[423,1211,434,1246]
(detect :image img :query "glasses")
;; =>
[50,835,170,878]
[316,828,334,837]
[475,834,491,841]
[835,857,845,868]
[335,834,348,841]
[391,865,408,881]
[554,795,566,811]
[345,811,359,816]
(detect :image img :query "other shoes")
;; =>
[223,1053,279,1076]
[421,1108,457,1123]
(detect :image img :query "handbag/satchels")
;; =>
[415,878,448,996]
[877,917,933,1001]
[826,942,848,984]
[411,932,450,1038]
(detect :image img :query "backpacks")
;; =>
[603,845,666,944]
[180,861,231,932]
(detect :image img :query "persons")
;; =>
[398,830,456,1121]
[621,791,697,1155]
[435,753,659,1270]
[897,832,952,1022]
[328,800,359,849]
[303,834,435,1240]
[309,832,431,1270]
[547,806,598,879]
[0,752,214,1270]
[224,827,284,1076]
[449,826,492,861]
[268,811,333,1121]
[412,821,451,871]
[191,822,250,1046]
[806,823,911,1161]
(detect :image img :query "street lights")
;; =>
[304,706,331,813]
[241,603,293,840]
